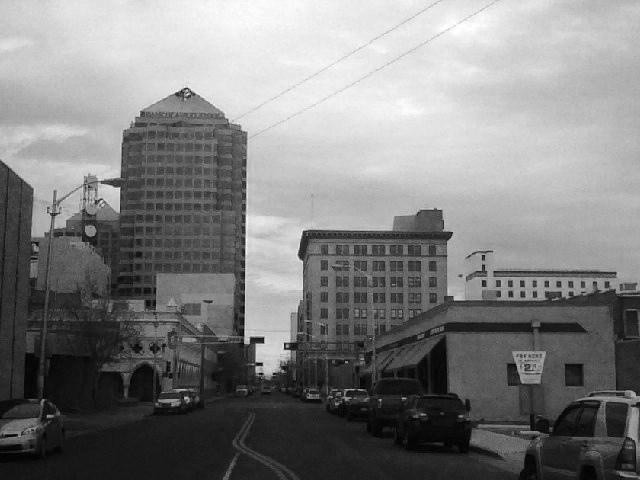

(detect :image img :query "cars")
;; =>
[326,386,474,454]
[235,382,322,401]
[152,387,200,416]
[0,396,69,461]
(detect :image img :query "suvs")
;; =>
[513,388,640,480]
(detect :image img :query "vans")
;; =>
[363,375,425,438]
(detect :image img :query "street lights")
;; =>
[297,331,322,344]
[329,264,377,386]
[304,319,329,396]
[35,177,129,397]
[176,298,215,387]
